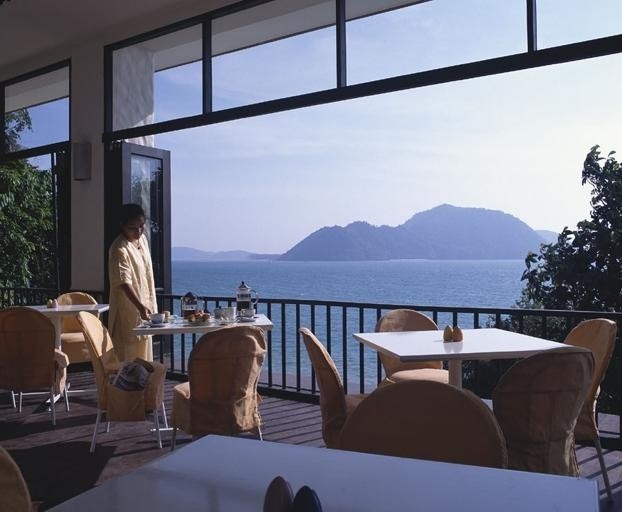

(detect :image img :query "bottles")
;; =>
[182,292,197,321]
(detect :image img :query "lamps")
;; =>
[70,141,92,185]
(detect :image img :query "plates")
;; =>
[143,322,170,328]
[183,320,217,326]
[236,315,261,322]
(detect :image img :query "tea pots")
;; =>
[236,281,259,310]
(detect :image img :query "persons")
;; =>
[109,202,157,366]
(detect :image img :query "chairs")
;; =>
[1,302,73,423]
[77,311,171,455]
[336,380,507,469]
[492,345,597,478]
[0,446,35,512]
[47,292,101,395]
[562,318,616,444]
[373,306,453,384]
[298,326,371,450]
[170,326,271,453]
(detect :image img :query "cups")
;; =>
[148,313,166,324]
[241,308,256,317]
